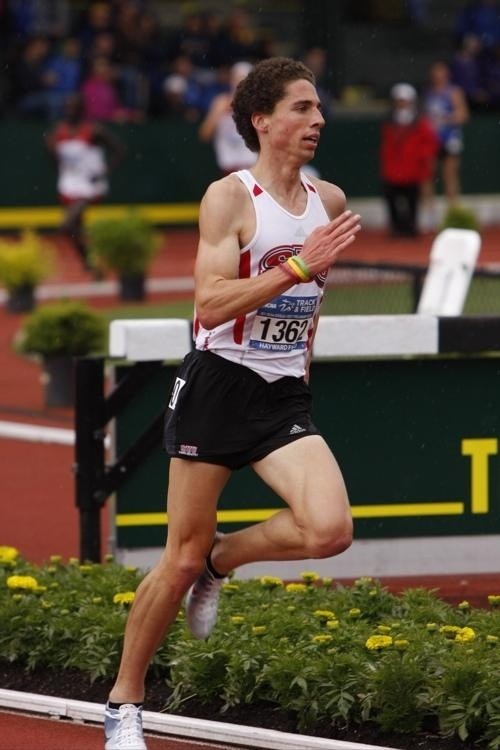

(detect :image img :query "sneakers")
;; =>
[101,700,150,750]
[185,530,230,642]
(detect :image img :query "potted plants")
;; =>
[0,231,59,313]
[11,304,110,408]
[91,215,165,301]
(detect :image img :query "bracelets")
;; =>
[278,254,315,284]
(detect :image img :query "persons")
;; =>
[193,61,259,180]
[1,1,499,121]
[378,81,438,235]
[103,54,363,750]
[43,92,121,283]
[414,55,473,233]
